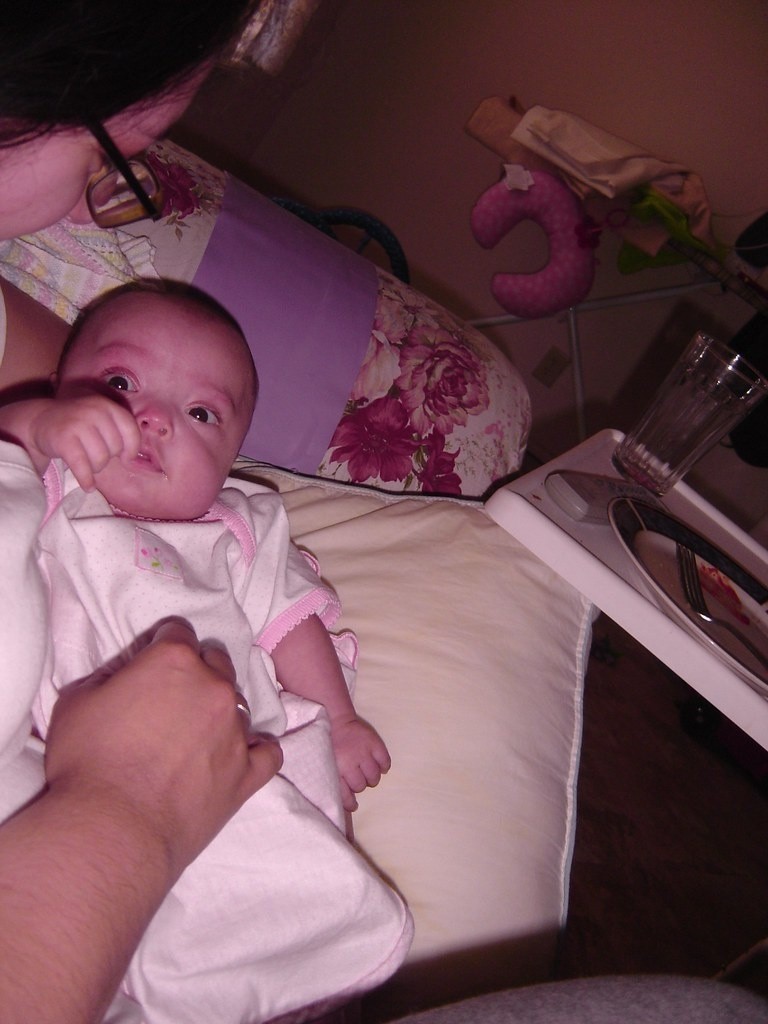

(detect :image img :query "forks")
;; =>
[676,542,768,672]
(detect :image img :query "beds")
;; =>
[0,100,598,1024]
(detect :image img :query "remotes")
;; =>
[546,470,671,524]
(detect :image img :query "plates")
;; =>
[608,497,768,698]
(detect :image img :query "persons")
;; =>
[0,0,415,1024]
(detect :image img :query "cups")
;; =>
[611,330,768,497]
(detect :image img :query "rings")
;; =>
[236,704,251,721]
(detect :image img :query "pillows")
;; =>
[0,113,533,502]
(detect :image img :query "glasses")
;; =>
[4,86,159,230]
